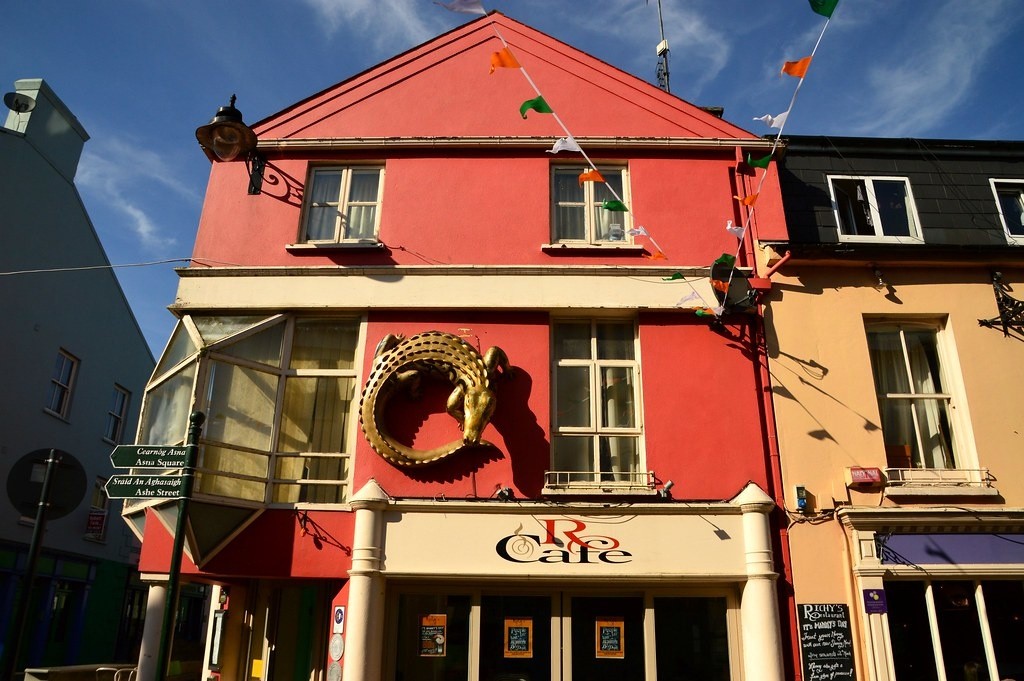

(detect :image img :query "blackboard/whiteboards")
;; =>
[796,602,856,681]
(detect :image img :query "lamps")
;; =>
[195,93,269,196]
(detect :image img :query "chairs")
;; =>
[885,444,913,468]
